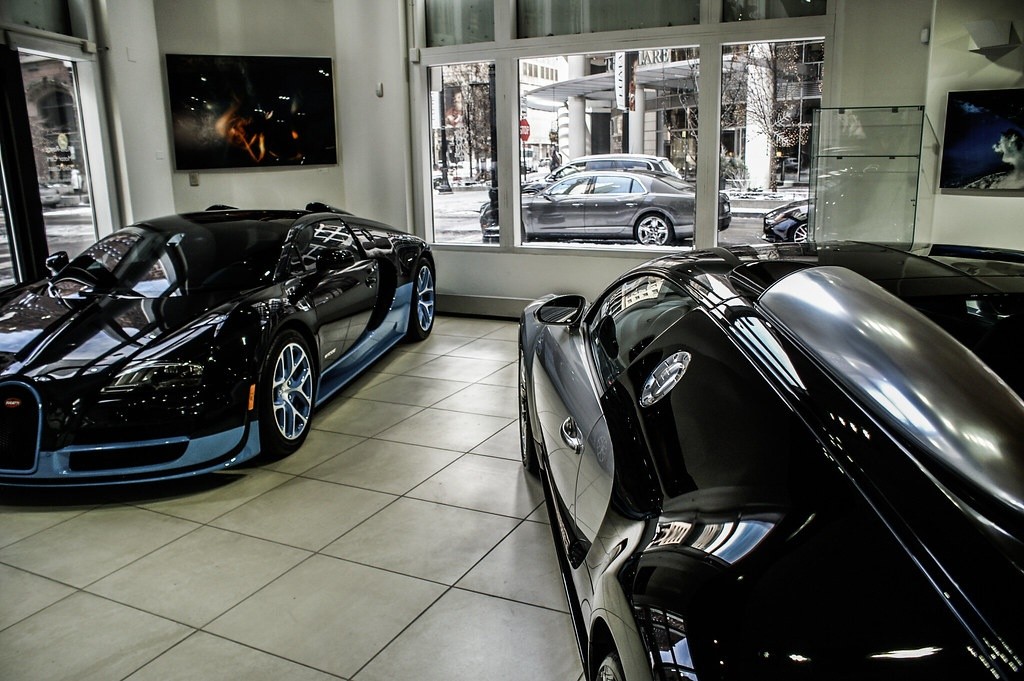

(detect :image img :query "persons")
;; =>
[446,89,463,125]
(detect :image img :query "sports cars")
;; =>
[0,202,437,492]
[518,244,1024,681]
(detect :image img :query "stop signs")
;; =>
[519,118,532,142]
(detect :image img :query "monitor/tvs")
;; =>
[166,54,337,170]
[939,89,1024,191]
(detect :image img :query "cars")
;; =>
[760,199,811,244]
[0,182,61,209]
[776,156,799,174]
[516,153,684,194]
[477,169,732,247]
